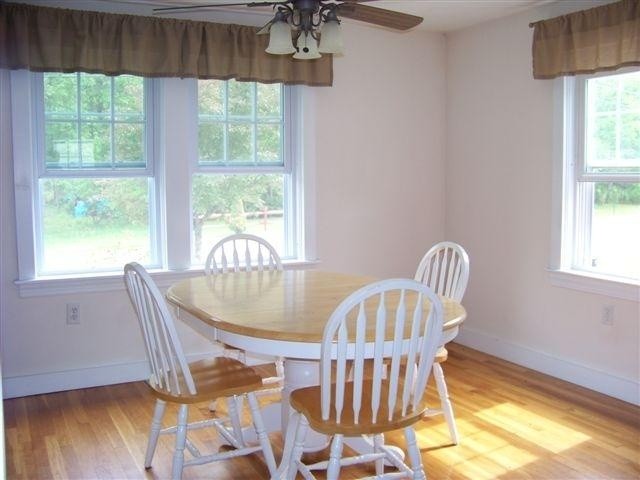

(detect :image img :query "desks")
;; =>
[166,265,468,479]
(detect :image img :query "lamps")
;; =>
[242,1,358,62]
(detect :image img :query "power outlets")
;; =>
[67,303,81,327]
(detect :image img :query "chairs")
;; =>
[120,258,276,480]
[206,233,286,451]
[381,239,469,447]
[275,279,448,480]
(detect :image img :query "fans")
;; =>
[142,0,428,33]
